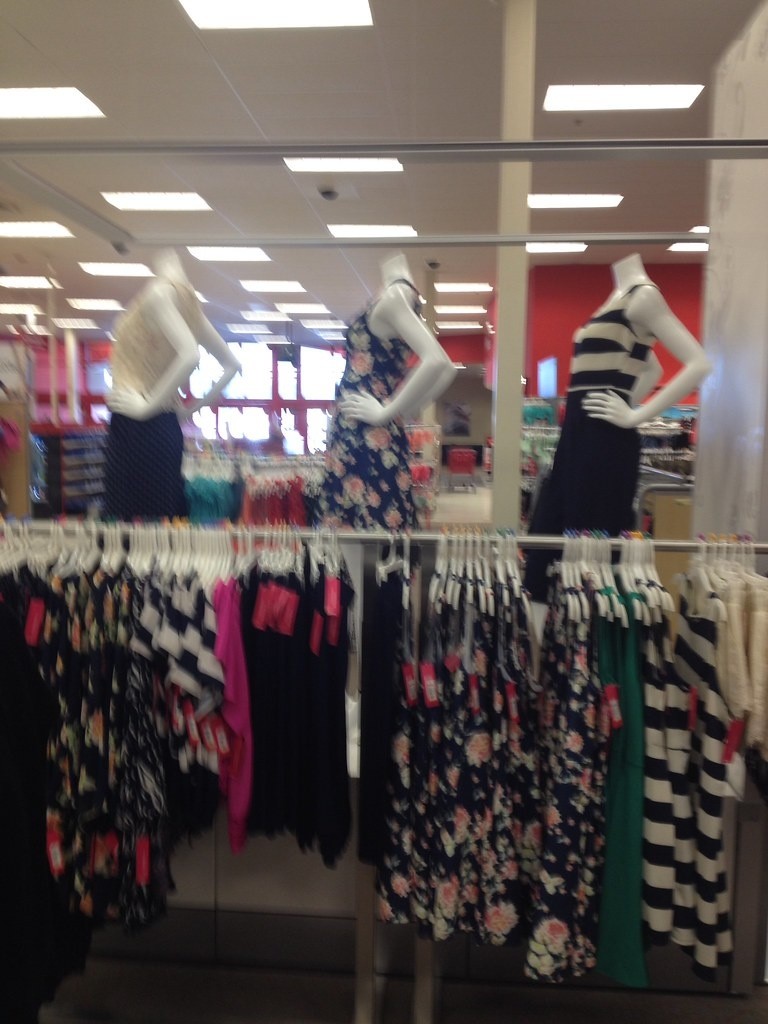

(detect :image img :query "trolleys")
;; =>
[447,449,479,494]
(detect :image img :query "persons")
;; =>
[519,251,712,643]
[101,248,242,521]
[319,254,458,532]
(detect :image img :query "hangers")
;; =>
[1,517,767,628]
